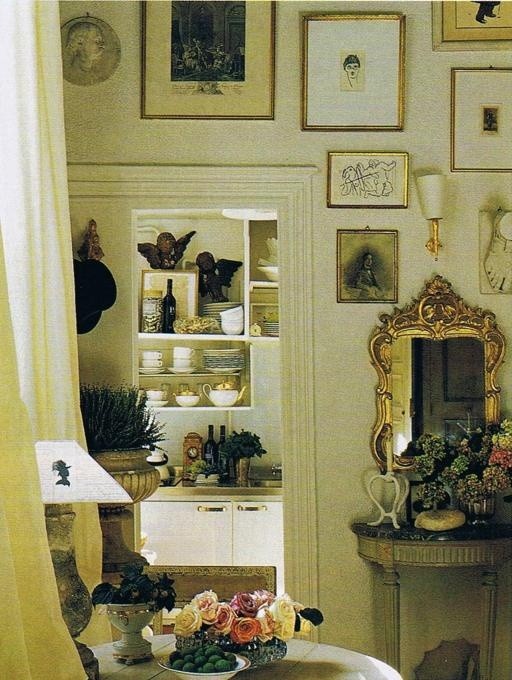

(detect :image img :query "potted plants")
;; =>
[218,429,267,486]
[79,381,170,515]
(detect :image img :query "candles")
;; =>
[385,437,393,474]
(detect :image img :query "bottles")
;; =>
[203,425,217,469]
[163,279,176,333]
[215,426,230,482]
[143,291,163,332]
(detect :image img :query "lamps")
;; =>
[35,440,134,638]
[416,174,447,260]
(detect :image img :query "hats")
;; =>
[74,258,116,334]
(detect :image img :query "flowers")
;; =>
[174,590,324,646]
[416,419,512,506]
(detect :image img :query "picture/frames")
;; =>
[451,67,512,174]
[431,0,511,51]
[302,14,405,132]
[328,151,410,208]
[140,1,276,120]
[337,229,399,304]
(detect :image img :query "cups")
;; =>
[172,346,195,360]
[146,390,165,400]
[174,360,194,368]
[271,461,283,480]
[143,359,161,368]
[142,351,162,361]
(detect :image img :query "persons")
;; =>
[352,251,381,297]
[343,53,365,88]
[77,220,104,262]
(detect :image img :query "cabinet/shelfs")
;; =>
[352,522,512,680]
[129,209,283,489]
[136,496,283,567]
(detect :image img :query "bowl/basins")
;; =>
[219,304,244,334]
[257,265,278,282]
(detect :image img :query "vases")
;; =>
[458,497,497,526]
[223,636,287,669]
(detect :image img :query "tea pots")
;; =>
[202,379,248,407]
[173,389,203,406]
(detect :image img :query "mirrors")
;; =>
[369,275,506,480]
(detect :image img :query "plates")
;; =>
[138,366,165,373]
[159,654,250,680]
[203,302,243,334]
[263,322,279,337]
[202,347,245,374]
[142,400,168,406]
[165,366,199,375]
